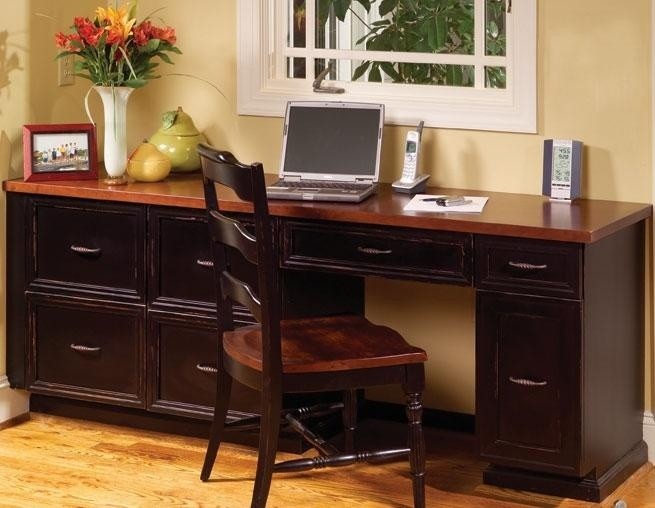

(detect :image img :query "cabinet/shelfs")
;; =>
[2,174,655,502]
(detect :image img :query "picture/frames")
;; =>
[23,124,99,182]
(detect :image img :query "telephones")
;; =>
[391,121,431,193]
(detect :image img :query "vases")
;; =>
[93,86,135,186]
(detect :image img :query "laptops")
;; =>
[266,99,385,203]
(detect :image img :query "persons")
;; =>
[40,141,78,163]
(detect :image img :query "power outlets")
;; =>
[58,47,77,87]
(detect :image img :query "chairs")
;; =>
[196,143,427,508]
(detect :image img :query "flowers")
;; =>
[38,0,236,140]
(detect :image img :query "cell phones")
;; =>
[437,193,473,206]
